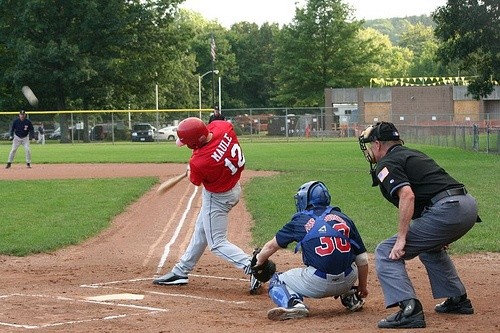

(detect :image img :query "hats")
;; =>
[20,109,25,115]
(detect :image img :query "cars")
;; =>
[0,126,91,142]
[152,125,179,142]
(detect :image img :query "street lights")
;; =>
[198,69,220,120]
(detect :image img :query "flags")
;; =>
[210,36,216,62]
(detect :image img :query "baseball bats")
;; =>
[154,171,189,195]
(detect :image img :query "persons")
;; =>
[154,117,262,293]
[6,110,34,169]
[360,121,481,328]
[208,106,226,123]
[36,123,45,145]
[251,181,368,321]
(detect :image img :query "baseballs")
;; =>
[29,96,38,106]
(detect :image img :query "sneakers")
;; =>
[244,263,262,295]
[378,299,426,328]
[434,296,474,315]
[152,271,189,286]
[339,285,365,311]
[266,299,309,321]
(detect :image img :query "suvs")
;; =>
[225,112,275,136]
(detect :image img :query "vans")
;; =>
[91,123,117,142]
[130,123,156,142]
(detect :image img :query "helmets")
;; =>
[176,117,209,150]
[293,180,331,212]
[360,121,400,143]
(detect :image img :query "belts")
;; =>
[314,266,353,280]
[425,186,468,206]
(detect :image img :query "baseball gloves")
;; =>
[249,248,276,282]
